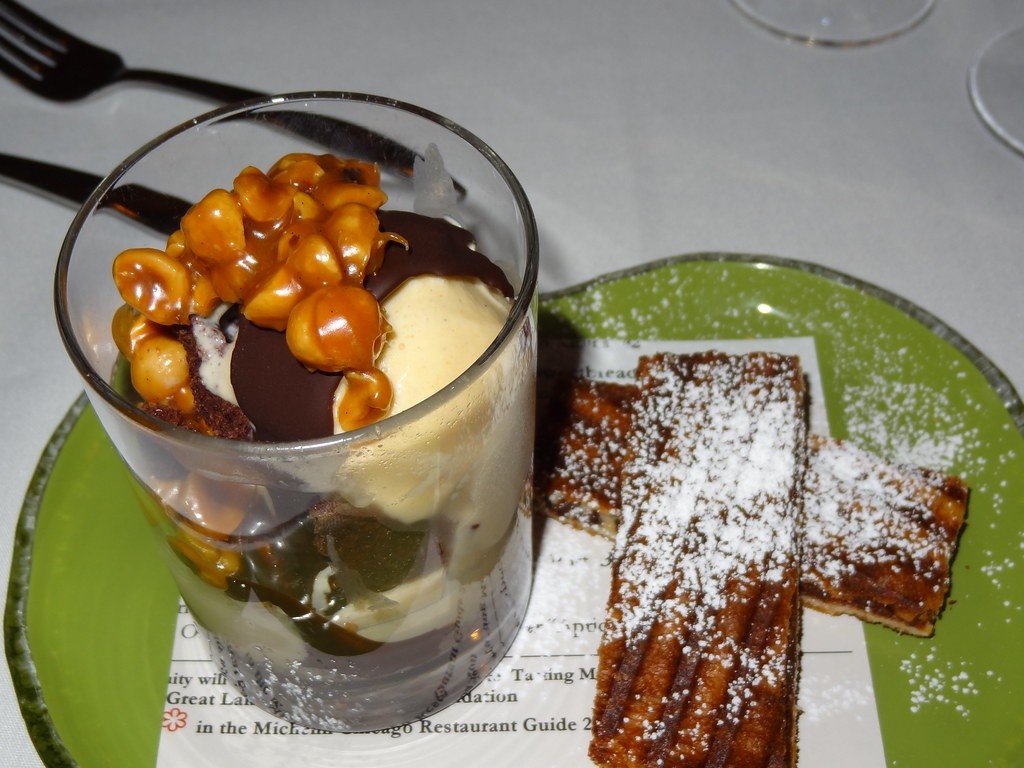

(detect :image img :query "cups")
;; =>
[53,89,538,732]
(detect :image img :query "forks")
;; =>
[0,0,469,200]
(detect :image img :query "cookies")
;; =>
[534,363,972,636]
[589,351,811,768]
